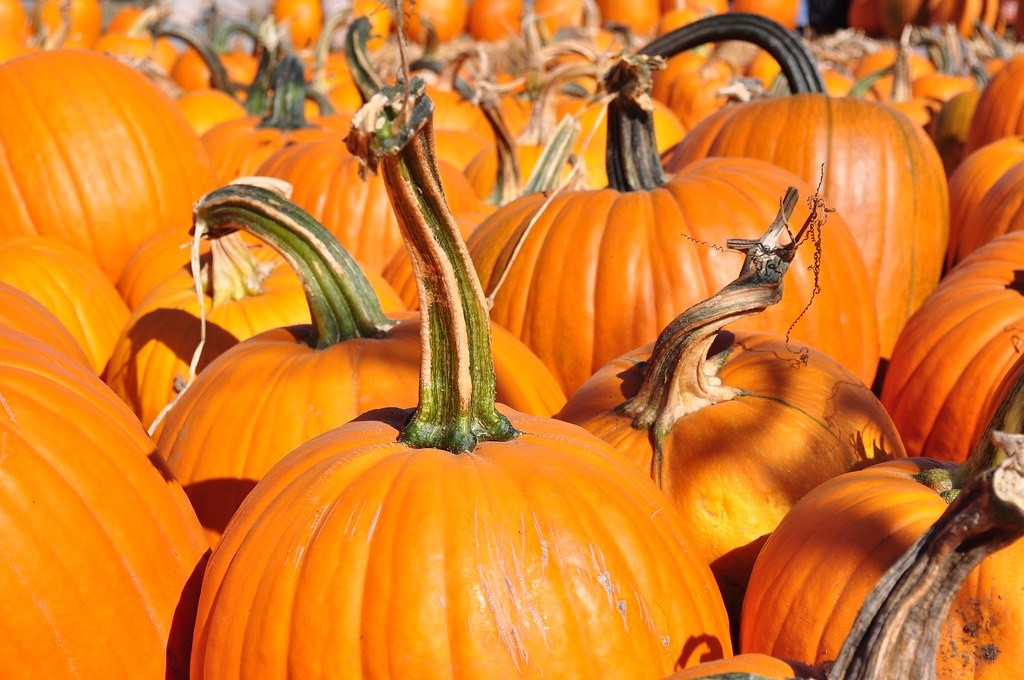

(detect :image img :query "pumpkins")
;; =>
[0,0,1024,680]
